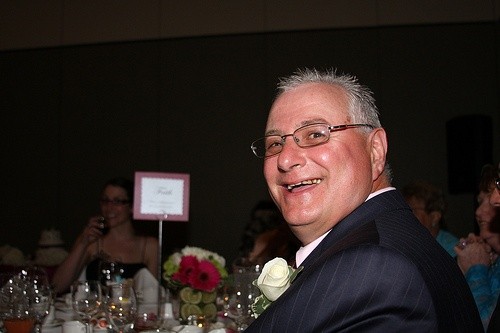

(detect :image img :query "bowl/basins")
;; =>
[3,317,36,333]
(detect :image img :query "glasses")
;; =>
[494,178,500,189]
[250,123,376,159]
[99,197,129,206]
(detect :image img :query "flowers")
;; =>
[163,246,229,293]
[250,257,304,319]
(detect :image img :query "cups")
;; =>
[172,324,203,333]
[62,322,87,333]
[64,293,72,307]
[42,307,55,323]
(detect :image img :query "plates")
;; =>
[55,304,75,313]
[36,318,65,327]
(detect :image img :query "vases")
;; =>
[176,287,221,325]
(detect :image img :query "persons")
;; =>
[50,177,158,295]
[244,68,484,333]
[401,181,460,257]
[453,139,500,333]
[486,162,500,333]
[234,200,302,274]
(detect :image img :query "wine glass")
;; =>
[0,258,267,333]
[91,215,111,258]
